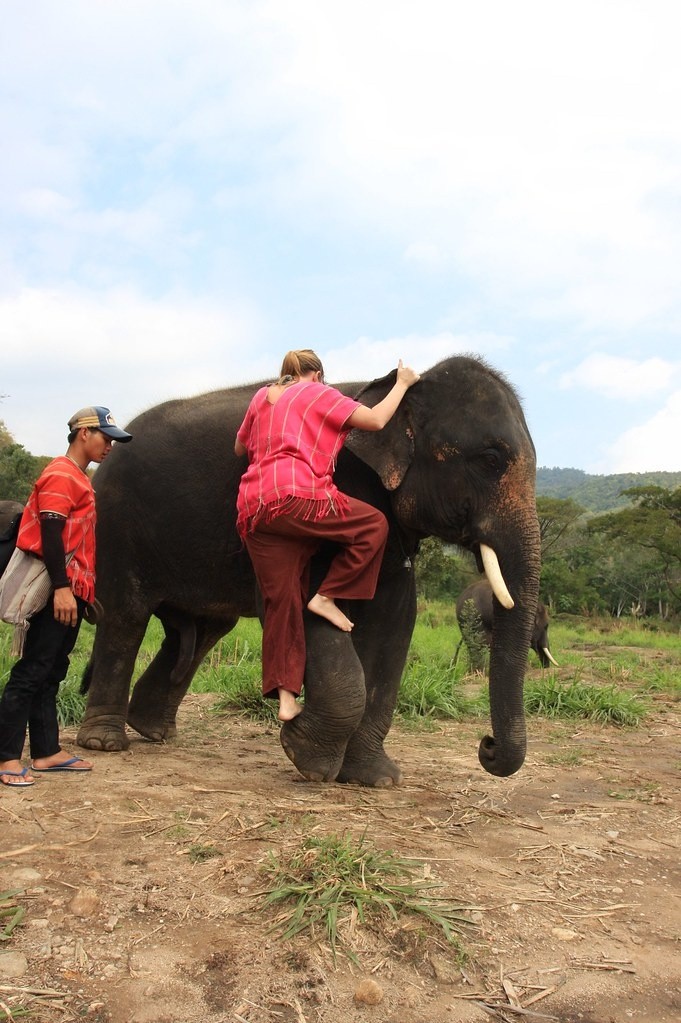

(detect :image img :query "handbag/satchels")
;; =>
[0,547,74,625]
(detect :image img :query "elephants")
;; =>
[0,349,545,790]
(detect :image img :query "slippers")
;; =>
[32,756,94,771]
[0,768,35,786]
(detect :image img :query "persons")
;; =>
[0,406,132,787]
[235,348,421,722]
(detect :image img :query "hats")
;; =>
[68,406,133,443]
